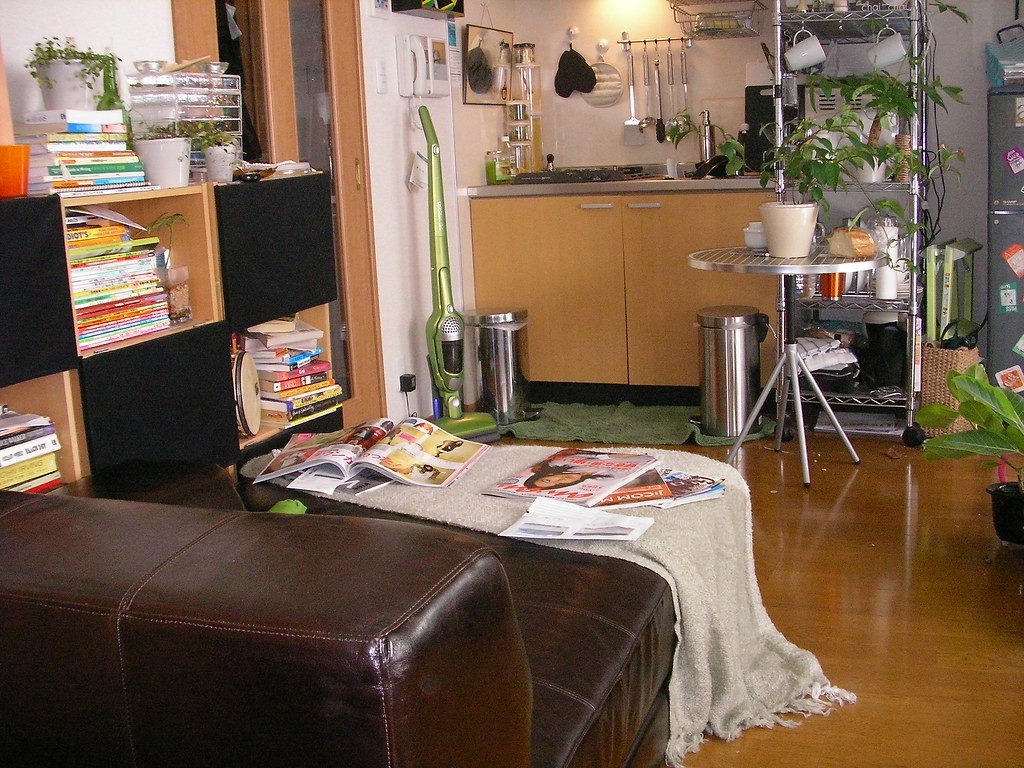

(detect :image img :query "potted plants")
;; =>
[914,360,1024,550]
[667,0,972,275]
[202,124,240,185]
[23,37,129,112]
[126,120,202,188]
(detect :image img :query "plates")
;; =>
[845,270,924,294]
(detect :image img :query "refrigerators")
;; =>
[985,85,1024,400]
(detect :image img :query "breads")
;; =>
[827,226,873,258]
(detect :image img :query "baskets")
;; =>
[920,341,980,438]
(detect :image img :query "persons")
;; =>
[435,440,464,458]
[384,464,434,476]
[524,453,652,490]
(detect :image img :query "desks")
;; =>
[686,247,880,489]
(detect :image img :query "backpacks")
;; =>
[787,333,860,395]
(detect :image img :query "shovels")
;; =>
[624,51,645,146]
[663,48,680,142]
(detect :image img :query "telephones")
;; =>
[397,32,427,96]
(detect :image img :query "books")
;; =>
[482,448,726,509]
[252,417,491,488]
[65,205,171,350]
[244,312,347,428]
[14,109,161,197]
[0,403,63,493]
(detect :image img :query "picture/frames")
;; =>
[463,24,514,105]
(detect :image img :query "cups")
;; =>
[0,144,31,198]
[820,272,844,301]
[783,30,827,72]
[894,271,911,283]
[866,28,907,69]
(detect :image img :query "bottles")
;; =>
[485,43,543,185]
[794,274,817,299]
[742,220,769,252]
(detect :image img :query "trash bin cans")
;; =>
[688,305,772,437]
[479,309,545,424]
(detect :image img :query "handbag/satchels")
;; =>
[856,338,891,389]
[983,25,1024,88]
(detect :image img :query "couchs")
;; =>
[0,453,737,768]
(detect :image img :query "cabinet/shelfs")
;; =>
[0,180,347,491]
[471,188,783,409]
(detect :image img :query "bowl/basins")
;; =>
[196,61,230,74]
[133,60,168,74]
